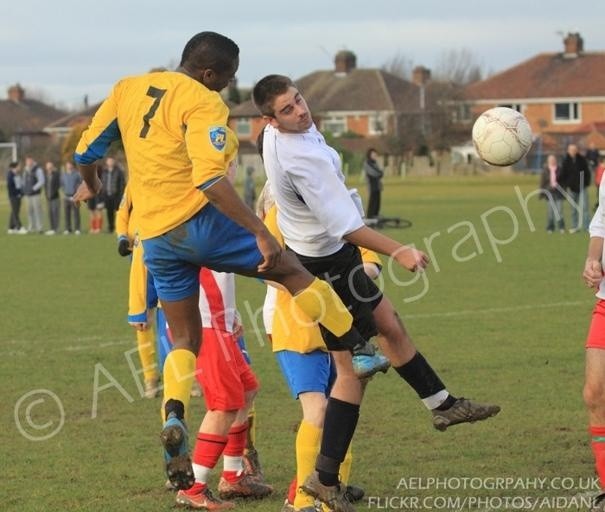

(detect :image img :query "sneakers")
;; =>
[288,470,364,512]
[548,228,578,234]
[432,397,500,432]
[88,228,102,234]
[218,448,273,499]
[176,483,235,512]
[351,344,391,379]
[161,418,196,490]
[6,226,81,235]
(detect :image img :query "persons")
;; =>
[582,170,605,508]
[592,159,605,210]
[0,30,502,512]
[586,143,599,168]
[561,143,590,235]
[538,155,565,234]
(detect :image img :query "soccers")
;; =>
[471,107,534,167]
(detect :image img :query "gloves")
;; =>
[118,240,132,256]
